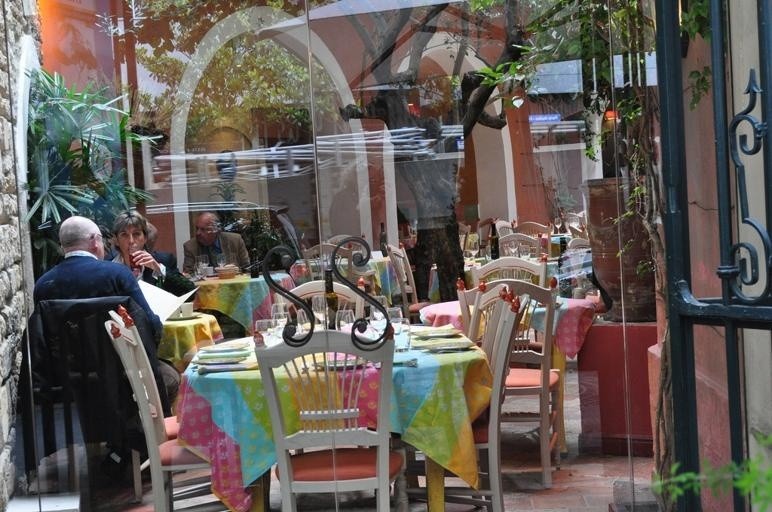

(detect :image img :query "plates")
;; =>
[190,342,251,367]
[167,316,198,322]
[410,327,479,353]
[312,358,364,370]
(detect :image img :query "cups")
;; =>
[504,241,531,261]
[225,264,239,274]
[369,295,412,353]
[193,253,226,282]
[255,303,311,348]
[335,308,355,331]
[169,308,180,318]
[180,302,194,317]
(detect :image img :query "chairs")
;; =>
[384,220,586,366]
[405,281,530,510]
[455,276,563,490]
[105,304,270,509]
[22,297,167,503]
[178,219,406,335]
[252,326,410,512]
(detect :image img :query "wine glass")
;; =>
[554,217,563,235]
[311,295,330,330]
[464,231,480,266]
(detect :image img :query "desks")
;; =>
[170,325,496,511]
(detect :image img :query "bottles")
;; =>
[322,269,340,329]
[557,237,573,299]
[378,222,388,258]
[490,223,500,260]
[248,244,260,279]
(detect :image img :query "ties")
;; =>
[208,245,217,268]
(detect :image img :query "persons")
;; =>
[21,216,181,489]
[183,212,252,275]
[111,209,196,302]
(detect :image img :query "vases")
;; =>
[588,178,654,321]
